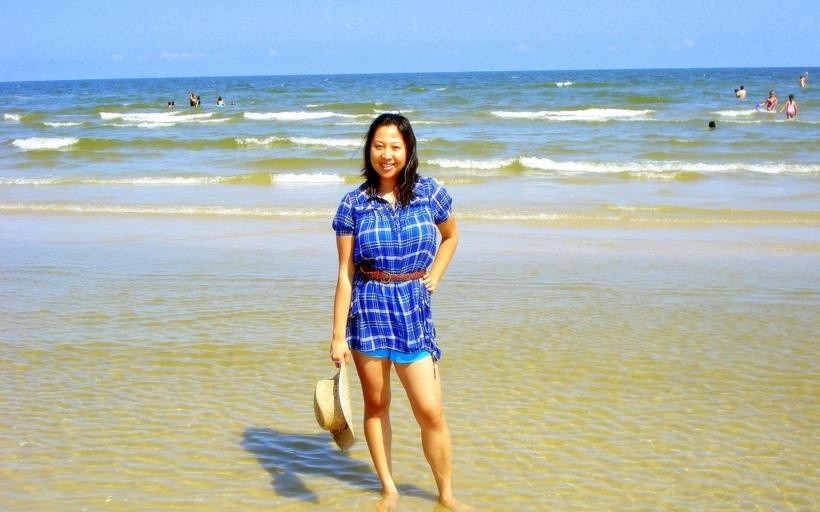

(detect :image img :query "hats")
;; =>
[313,362,355,451]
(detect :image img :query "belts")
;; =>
[359,265,426,284]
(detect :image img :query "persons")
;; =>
[765,89,777,111]
[734,89,740,98]
[329,114,470,511]
[217,96,223,105]
[168,91,201,108]
[780,94,798,120]
[736,86,746,99]
[800,76,807,87]
